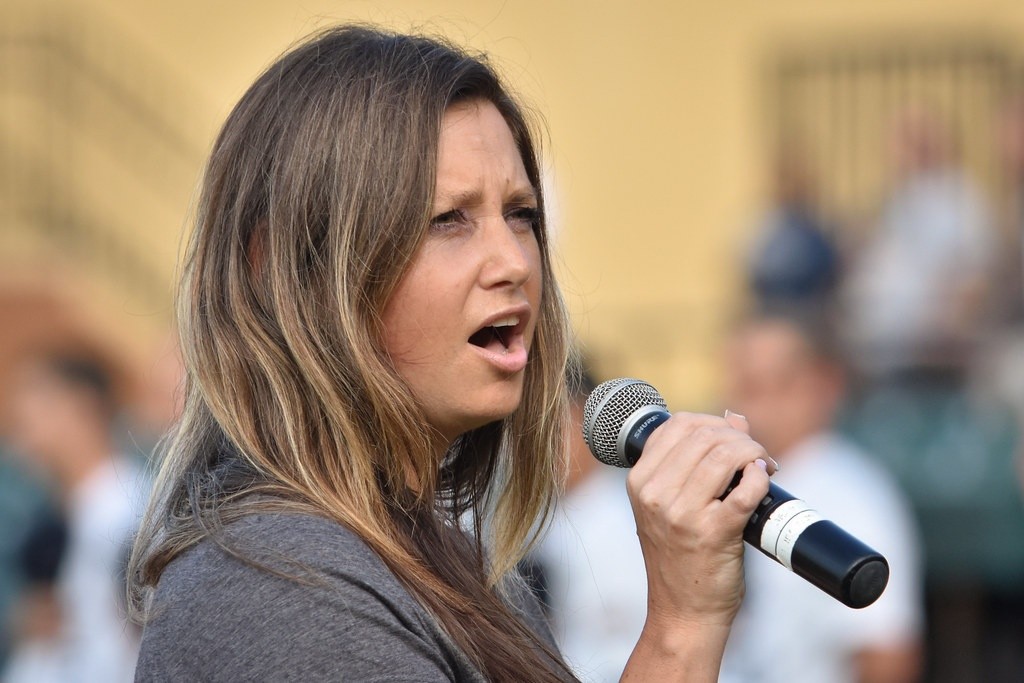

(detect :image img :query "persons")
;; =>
[126,24,778,683]
[0,266,922,683]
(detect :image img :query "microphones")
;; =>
[581,377,889,610]
